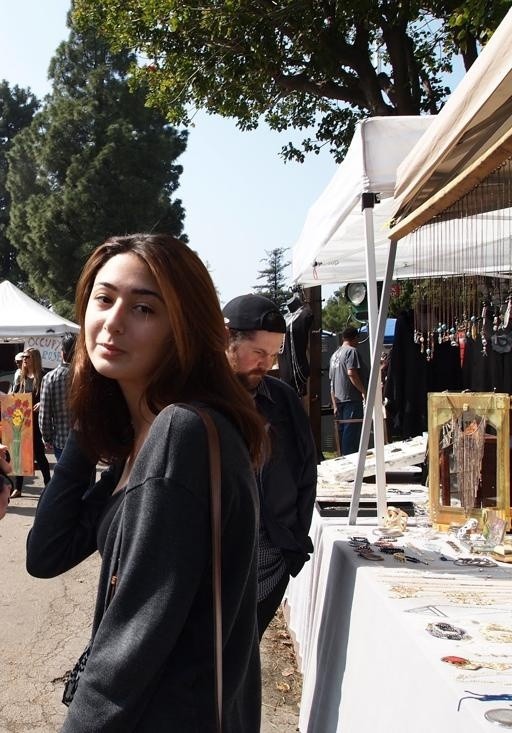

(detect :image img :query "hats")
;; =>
[343,326,361,338]
[222,293,286,333]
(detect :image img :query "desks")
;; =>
[281,462,463,658]
[299,526,512,733]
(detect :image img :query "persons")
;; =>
[329,326,366,454]
[219,293,318,647]
[0,333,96,520]
[25,229,269,733]
